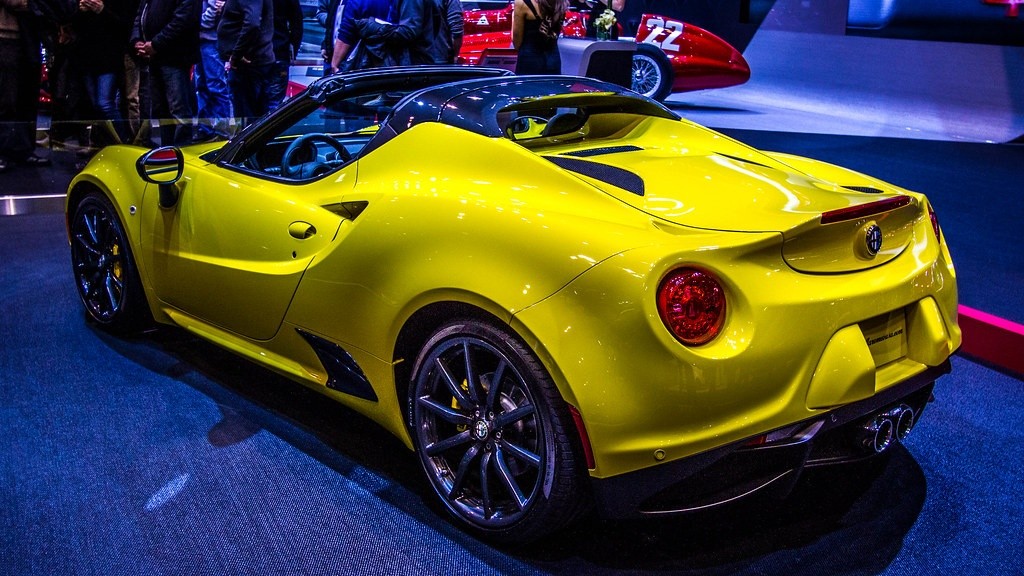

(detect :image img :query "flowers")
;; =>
[592,9,617,30]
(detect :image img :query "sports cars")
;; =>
[456,0,751,105]
[63,61,962,554]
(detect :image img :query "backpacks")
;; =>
[537,6,561,44]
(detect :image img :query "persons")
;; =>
[128,0,197,149]
[315,0,464,75]
[0,0,130,169]
[514,0,570,75]
[188,0,304,144]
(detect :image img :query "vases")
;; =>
[597,28,608,41]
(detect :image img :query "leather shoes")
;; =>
[25,154,52,168]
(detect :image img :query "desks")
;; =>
[557,37,638,90]
